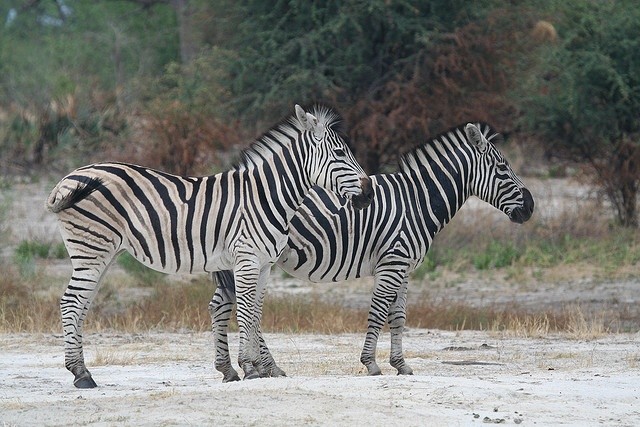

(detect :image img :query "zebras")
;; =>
[209,121,534,382]
[46,104,375,388]
